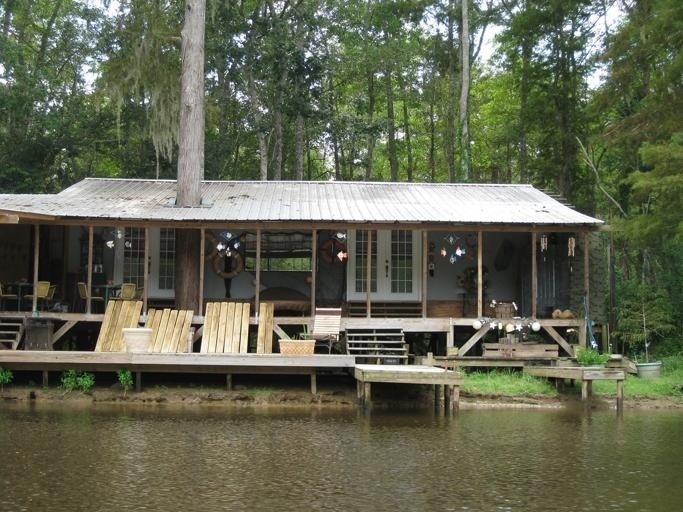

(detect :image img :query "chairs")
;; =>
[76,281,145,312]
[298,306,342,354]
[0,281,58,311]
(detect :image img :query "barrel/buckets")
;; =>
[122,327,153,354]
[636,360,661,379]
[495,302,517,317]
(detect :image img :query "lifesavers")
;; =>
[212,249,243,279]
[320,238,346,265]
[463,269,485,293]
[204,233,219,262]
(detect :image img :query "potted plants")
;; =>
[575,347,610,368]
